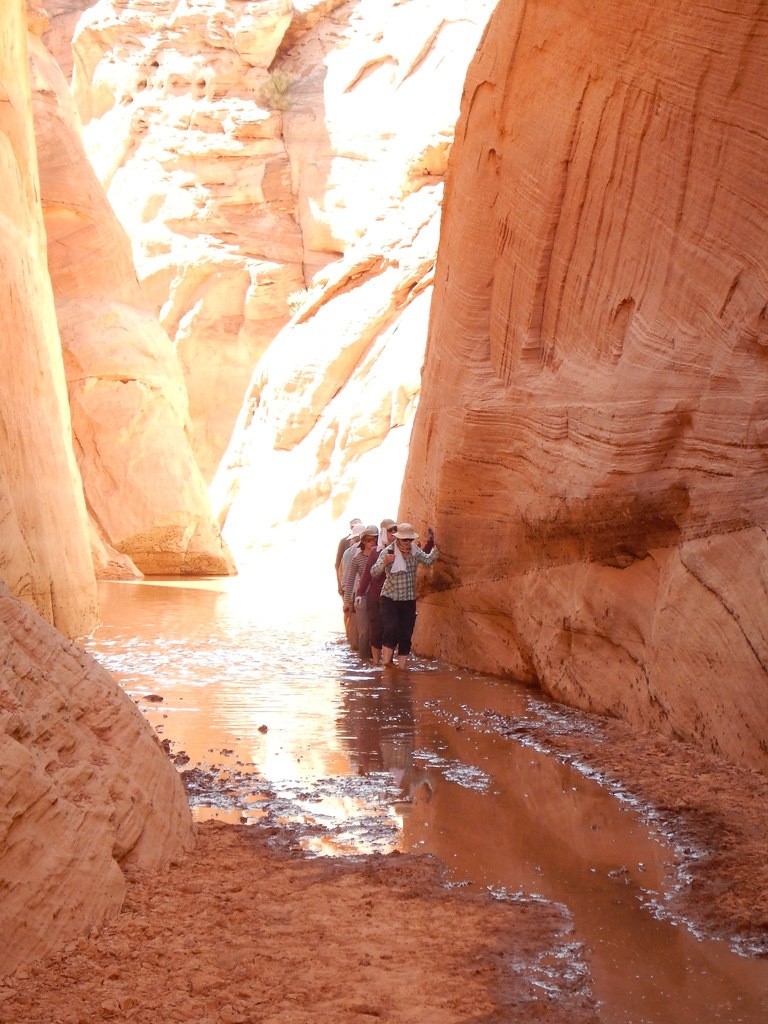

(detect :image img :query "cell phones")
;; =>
[388,550,395,563]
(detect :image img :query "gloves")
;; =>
[354,595,362,607]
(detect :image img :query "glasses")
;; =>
[386,527,397,532]
[402,539,415,542]
[367,538,377,542]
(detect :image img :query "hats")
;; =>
[361,525,379,536]
[349,518,363,527]
[346,523,366,541]
[391,522,419,539]
[380,518,397,530]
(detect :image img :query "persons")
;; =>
[370,523,438,669]
[334,517,434,663]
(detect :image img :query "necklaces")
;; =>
[397,542,410,552]
